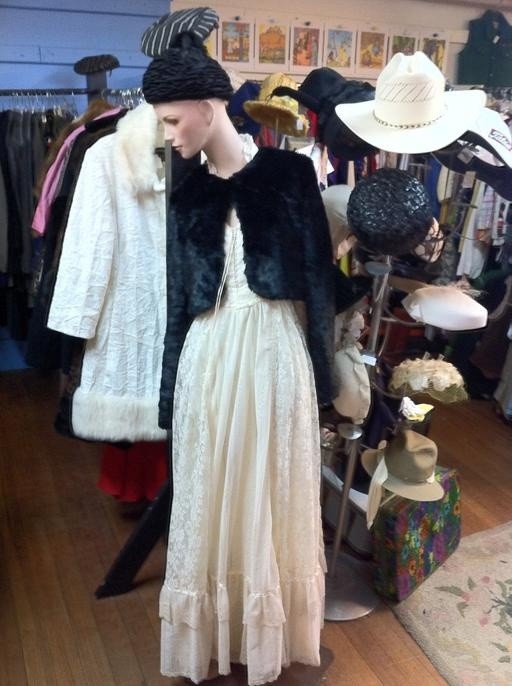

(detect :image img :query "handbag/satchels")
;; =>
[369,463,465,604]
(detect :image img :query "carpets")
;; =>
[382,519,512,686]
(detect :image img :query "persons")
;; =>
[292,30,413,68]
[139,30,339,686]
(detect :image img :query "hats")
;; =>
[329,262,377,318]
[359,426,449,503]
[333,49,489,156]
[329,340,374,427]
[264,63,382,161]
[386,354,473,406]
[427,105,512,202]
[139,29,237,105]
[318,166,446,264]
[399,285,491,332]
[241,69,310,139]
